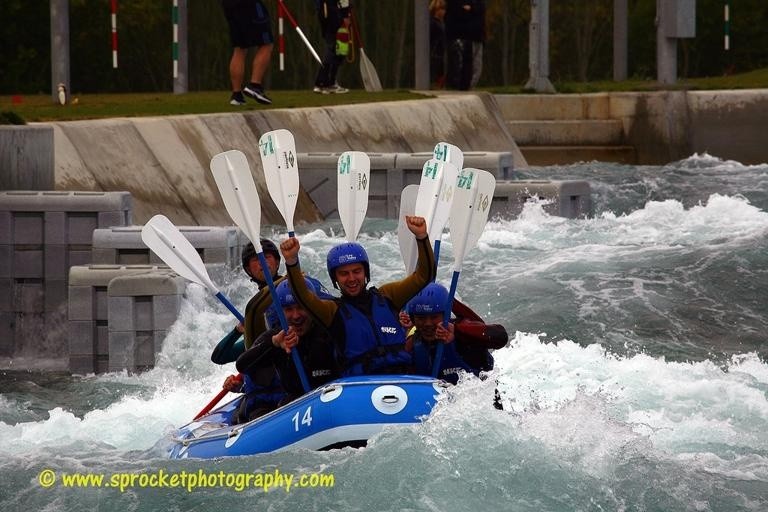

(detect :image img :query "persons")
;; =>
[220,1,274,104]
[222,373,244,396]
[432,0,486,91]
[210,324,244,368]
[278,215,437,379]
[239,240,283,343]
[312,0,359,96]
[237,277,339,424]
[402,285,507,380]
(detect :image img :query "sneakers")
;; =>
[230,92,247,105]
[313,85,322,92]
[323,83,350,94]
[244,82,273,106]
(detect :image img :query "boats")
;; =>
[167,374,453,460]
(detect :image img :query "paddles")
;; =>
[259,128,300,240]
[210,149,312,395]
[396,183,419,275]
[140,215,244,325]
[431,168,496,378]
[350,7,383,93]
[337,151,371,244]
[431,141,464,264]
[416,158,457,242]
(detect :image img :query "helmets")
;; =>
[327,242,371,288]
[241,238,281,277]
[276,277,317,305]
[405,282,451,334]
